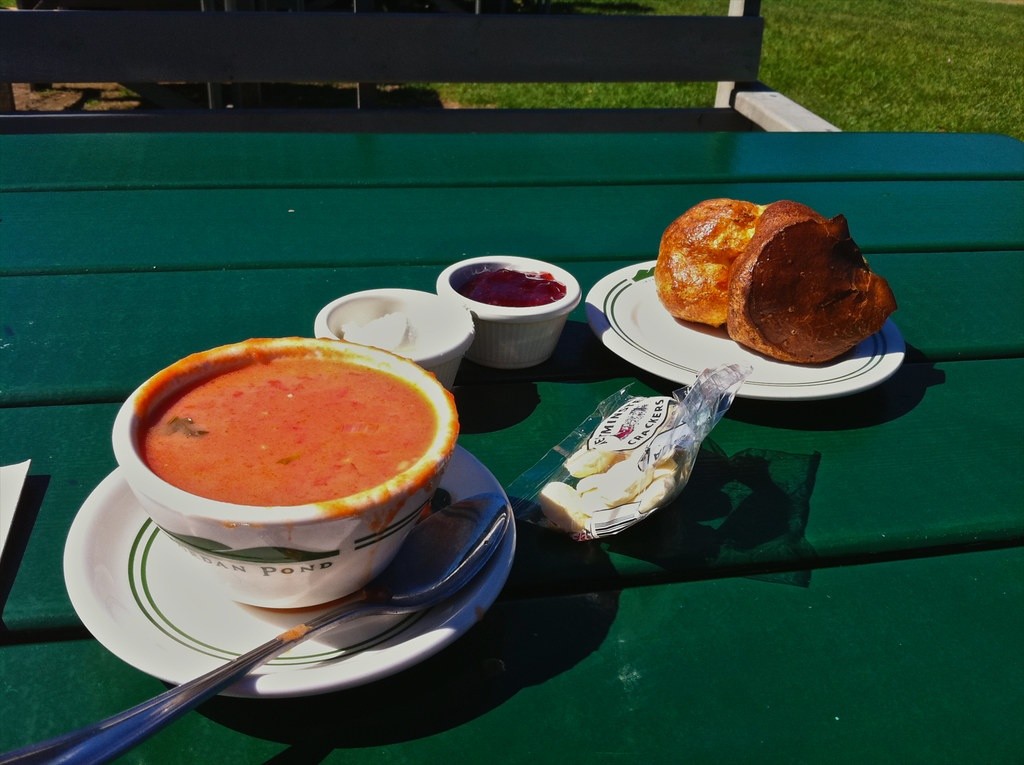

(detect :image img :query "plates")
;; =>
[583,259,906,401]
[62,440,516,698]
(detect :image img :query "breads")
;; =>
[653,198,772,328]
[726,200,899,365]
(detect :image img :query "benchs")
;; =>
[2,0,841,132]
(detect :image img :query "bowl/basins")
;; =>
[313,287,477,392]
[436,255,582,368]
[113,335,461,609]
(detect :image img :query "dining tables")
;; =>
[1,132,1022,762]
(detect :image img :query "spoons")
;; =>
[0,490,511,764]
